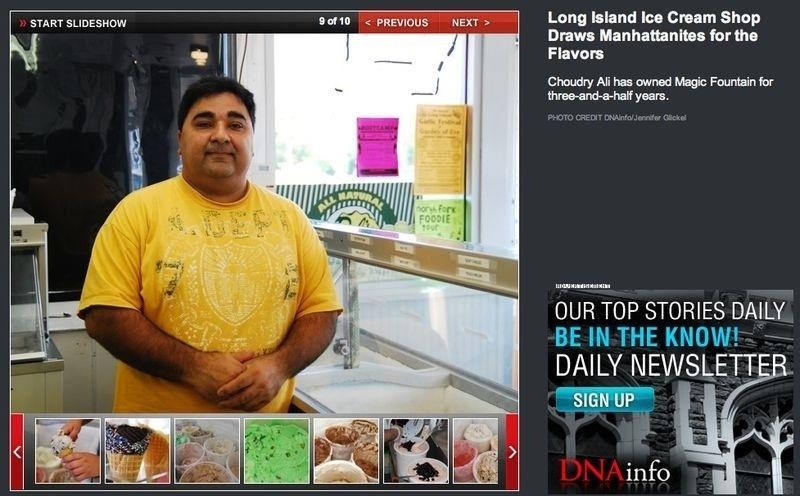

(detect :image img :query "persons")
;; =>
[58,419,100,483]
[77,73,343,415]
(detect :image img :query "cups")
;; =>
[175,422,240,484]
[313,418,499,485]
[35,446,80,483]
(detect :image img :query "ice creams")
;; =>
[50,430,76,459]
[105,419,151,483]
[454,424,498,484]
[393,435,449,483]
